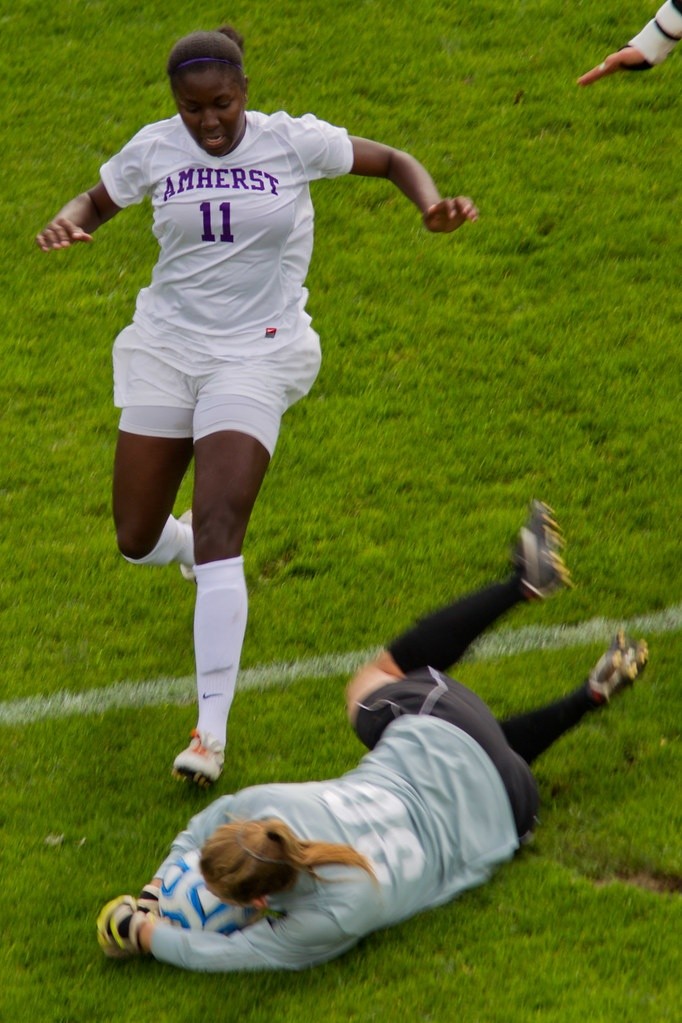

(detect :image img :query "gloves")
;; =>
[137,884,160,919]
[96,896,145,960]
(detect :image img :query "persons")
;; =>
[577,0,682,86]
[33,26,479,790]
[95,501,649,970]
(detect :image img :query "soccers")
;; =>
[157,848,262,939]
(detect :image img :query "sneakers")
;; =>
[177,509,196,581]
[587,631,650,703]
[509,499,573,602]
[171,729,225,790]
